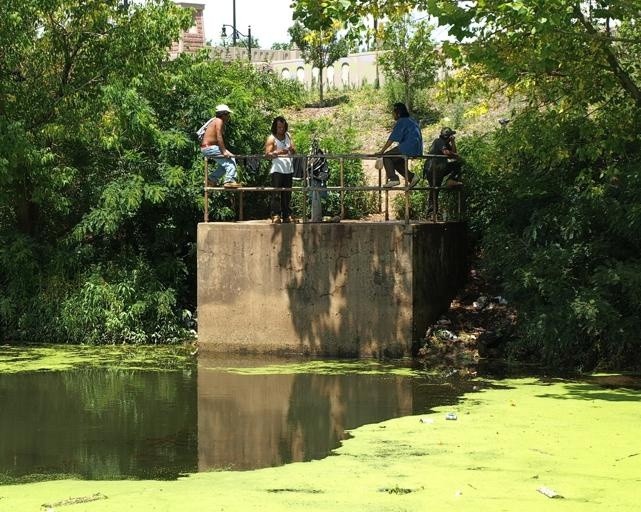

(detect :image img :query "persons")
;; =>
[425,127,465,222]
[265,116,298,224]
[377,102,421,192]
[202,104,242,189]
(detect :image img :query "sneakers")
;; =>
[405,175,422,193]
[382,179,400,189]
[447,179,464,187]
[205,180,244,188]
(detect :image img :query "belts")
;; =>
[200,142,214,149]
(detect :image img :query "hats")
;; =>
[440,127,458,138]
[215,103,234,114]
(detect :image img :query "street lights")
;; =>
[221,23,252,64]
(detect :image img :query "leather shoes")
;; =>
[272,213,300,224]
